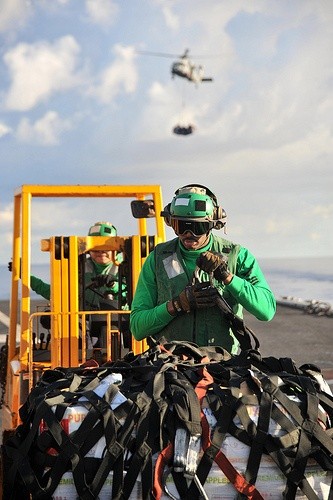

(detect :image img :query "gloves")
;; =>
[173,272,218,315]
[196,251,230,281]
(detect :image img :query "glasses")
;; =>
[170,217,215,237]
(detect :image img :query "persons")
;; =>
[9,221,130,310]
[130,184,276,354]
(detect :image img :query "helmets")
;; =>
[88,221,118,236]
[160,184,228,235]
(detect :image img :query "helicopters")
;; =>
[139,47,214,89]
